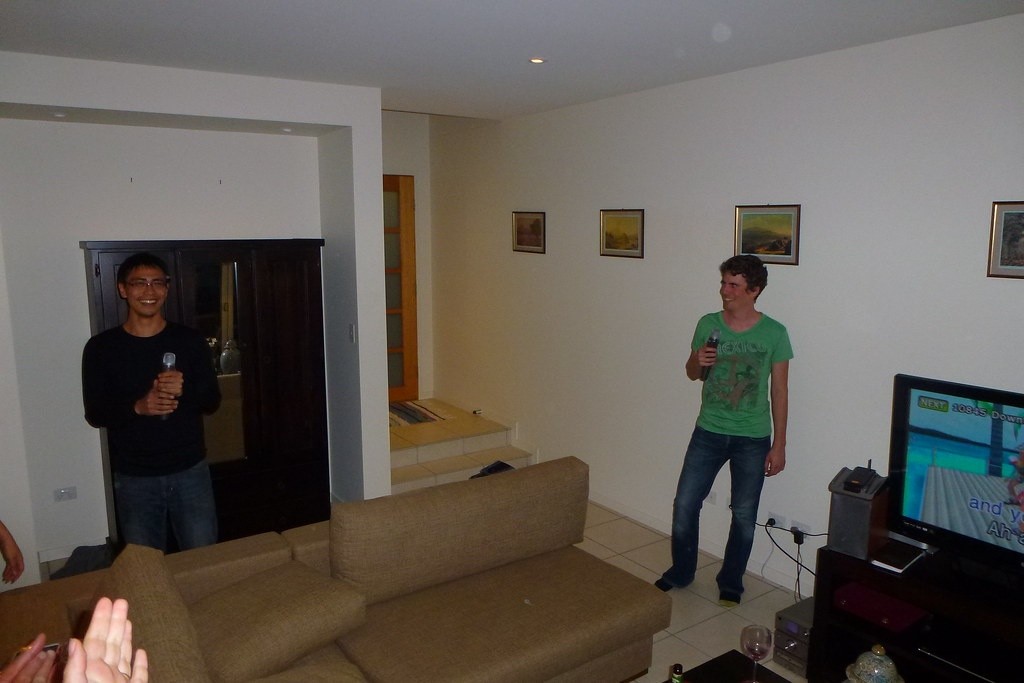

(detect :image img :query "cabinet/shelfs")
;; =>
[807,546,1023,683]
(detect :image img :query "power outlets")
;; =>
[766,510,809,538]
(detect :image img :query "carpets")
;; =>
[389,399,445,427]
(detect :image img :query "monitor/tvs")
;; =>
[885,373,1024,569]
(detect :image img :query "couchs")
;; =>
[0,457,672,683]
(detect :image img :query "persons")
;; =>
[83,253,221,553]
[0,597,149,683]
[0,520,24,584]
[657,258,794,607]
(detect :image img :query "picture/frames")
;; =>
[599,208,645,259]
[511,211,546,254]
[734,204,801,266]
[987,201,1024,280]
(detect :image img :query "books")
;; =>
[871,541,924,573]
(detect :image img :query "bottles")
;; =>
[841,645,906,683]
[220,340,239,374]
[671,662,683,683]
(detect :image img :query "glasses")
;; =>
[124,279,167,290]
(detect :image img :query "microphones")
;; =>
[699,332,721,381]
[161,352,176,419]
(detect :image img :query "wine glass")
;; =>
[741,624,771,683]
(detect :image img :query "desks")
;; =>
[663,648,792,683]
